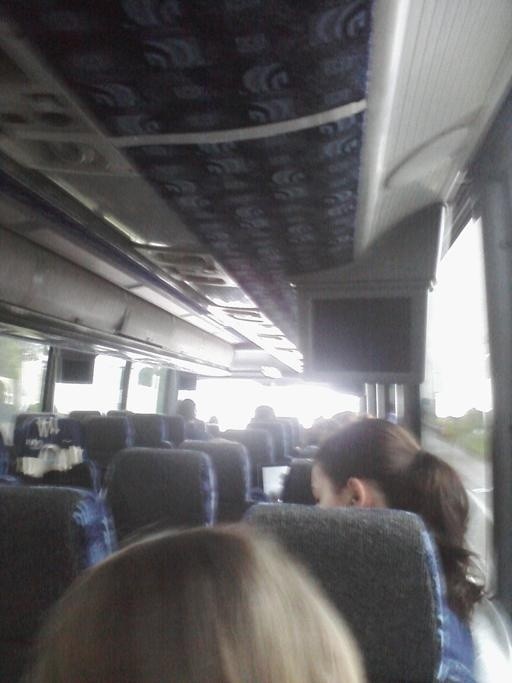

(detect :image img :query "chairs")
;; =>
[0,410,449,683]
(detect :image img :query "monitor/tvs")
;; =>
[304,290,428,383]
[56,351,95,384]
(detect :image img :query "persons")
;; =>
[254,404,276,422]
[310,417,490,621]
[179,397,200,423]
[23,523,368,680]
